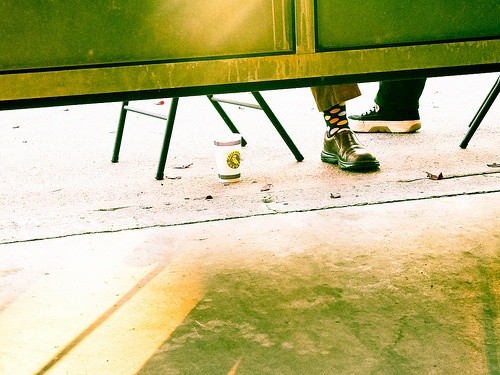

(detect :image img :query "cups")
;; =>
[214,133,242,182]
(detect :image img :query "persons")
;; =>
[308,82,379,172]
[346,78,427,133]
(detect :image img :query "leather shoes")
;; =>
[321,129,380,170]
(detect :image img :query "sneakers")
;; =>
[347,102,421,133]
